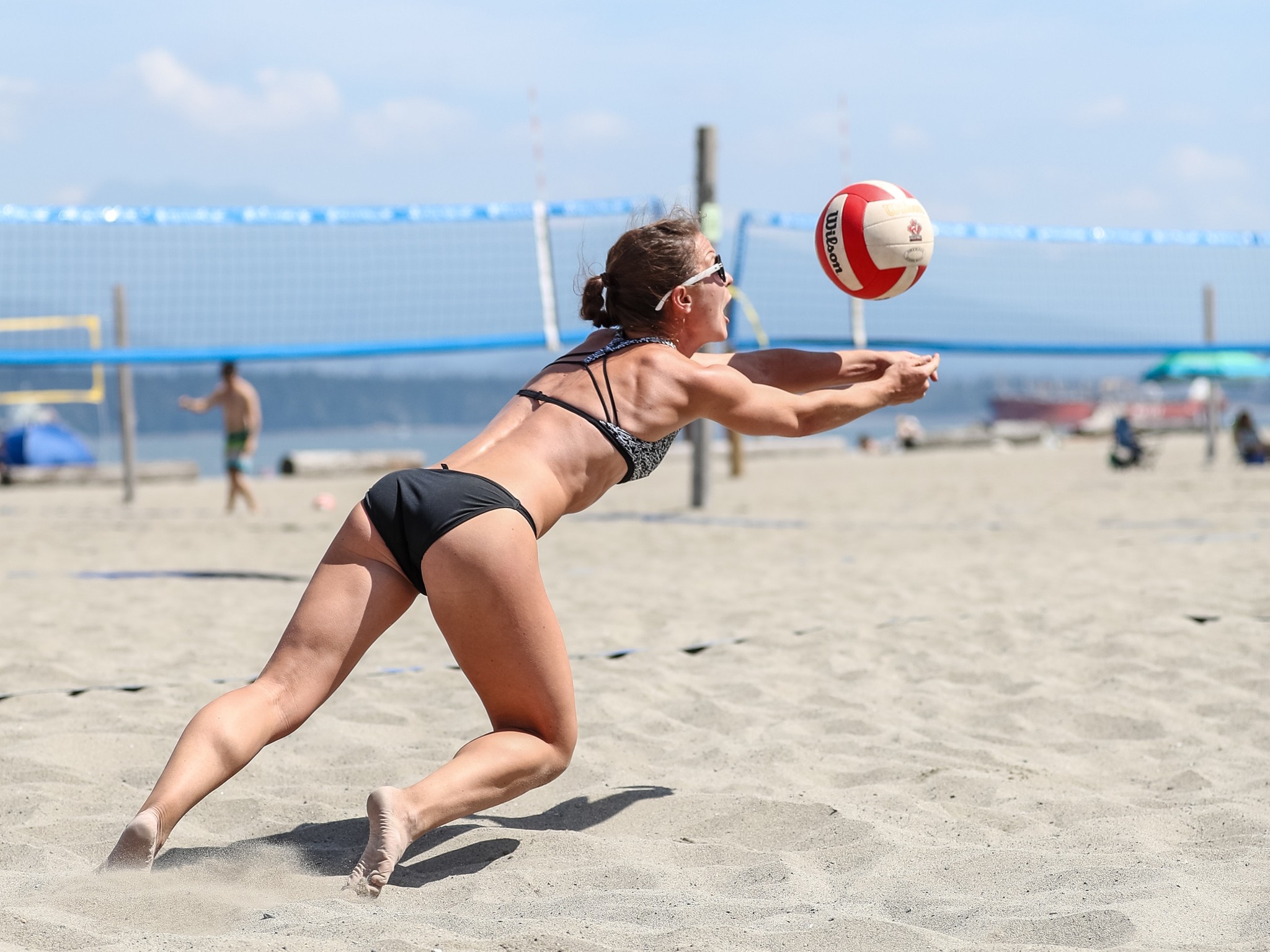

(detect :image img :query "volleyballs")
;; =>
[815,180,934,301]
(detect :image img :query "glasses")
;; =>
[655,254,727,311]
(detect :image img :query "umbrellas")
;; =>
[1141,350,1270,461]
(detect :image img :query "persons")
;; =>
[94,210,941,901]
[1235,410,1270,465]
[178,359,262,511]
[1109,416,1149,469]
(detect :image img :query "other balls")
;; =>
[309,494,336,511]
